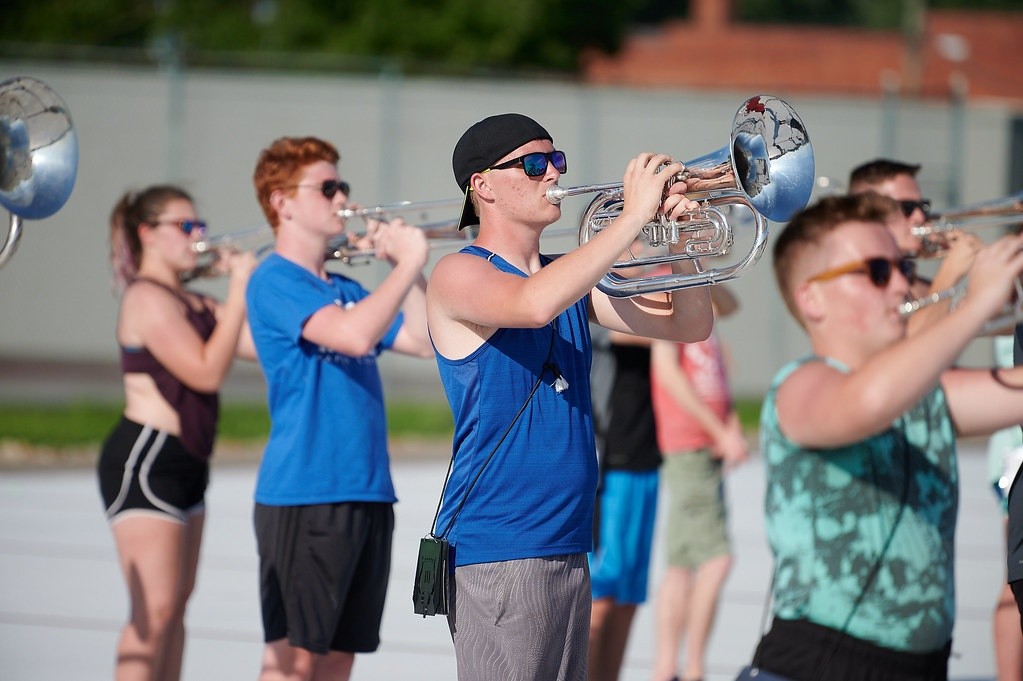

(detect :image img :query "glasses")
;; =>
[276,179,350,201]
[808,255,917,289]
[896,200,930,219]
[470,150,567,191]
[149,220,206,235]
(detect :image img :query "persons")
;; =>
[751,159,1023,681]
[425,113,714,681]
[94,183,257,681]
[576,198,750,681]
[244,136,436,681]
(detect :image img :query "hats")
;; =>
[452,113,553,231]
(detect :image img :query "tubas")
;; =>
[543,93,820,296]
[0,73,81,262]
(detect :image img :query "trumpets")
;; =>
[196,238,267,274]
[903,278,1021,343]
[338,200,478,255]
[924,192,1023,236]
